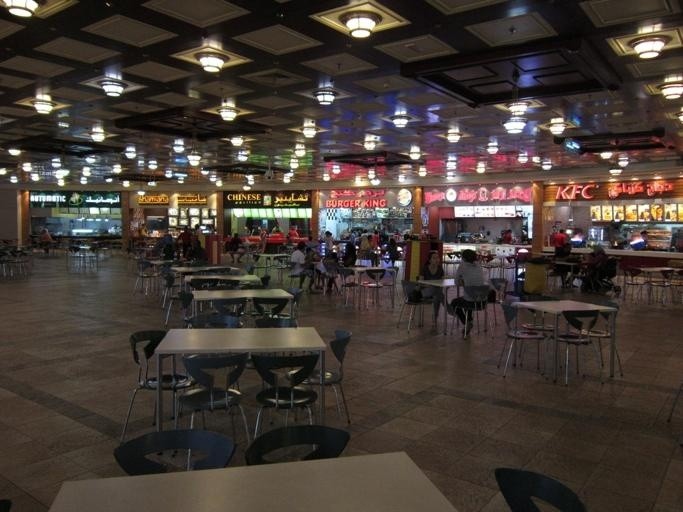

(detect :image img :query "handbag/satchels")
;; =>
[408,290,422,303]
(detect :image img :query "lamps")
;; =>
[29,98,59,116]
[2,0,38,18]
[340,10,384,40]
[216,106,240,122]
[391,114,413,128]
[656,79,682,101]
[631,35,671,60]
[311,87,341,109]
[192,51,230,74]
[506,101,532,117]
[98,79,129,99]
[1,119,681,190]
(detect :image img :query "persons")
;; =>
[420,227,649,334]
[40,228,53,254]
[222,226,300,266]
[290,225,412,292]
[149,224,211,266]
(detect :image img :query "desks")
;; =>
[44,451,460,512]
[154,325,329,434]
[516,300,618,384]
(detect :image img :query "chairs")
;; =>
[492,466,589,512]
[574,295,626,379]
[118,329,191,444]
[301,330,356,425]
[0,233,108,278]
[175,349,254,447]
[496,298,542,375]
[245,422,352,467]
[129,232,682,339]
[247,350,319,439]
[560,307,605,386]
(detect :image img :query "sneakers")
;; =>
[463,320,473,336]
